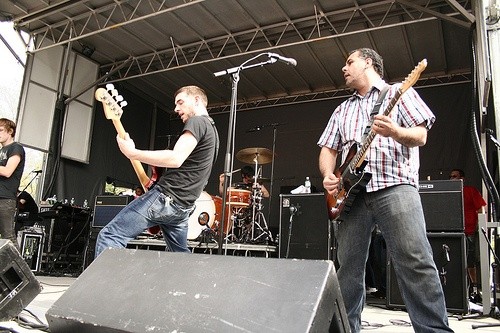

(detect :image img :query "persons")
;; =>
[15,188,39,238]
[219,166,270,244]
[0,118,25,255]
[94,85,219,267]
[316,47,454,333]
[448,168,487,293]
[134,187,145,197]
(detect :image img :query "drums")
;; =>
[186,191,233,241]
[226,188,252,209]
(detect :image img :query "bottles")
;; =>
[71,197,75,206]
[84,200,89,208]
[304,176,311,193]
[52,194,58,204]
[60,197,69,205]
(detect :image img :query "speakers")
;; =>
[385,232,471,315]
[93,205,128,228]
[84,227,101,270]
[279,193,340,271]
[44,246,351,333]
[0,237,43,322]
[417,179,465,232]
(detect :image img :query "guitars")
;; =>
[324,58,427,223]
[94,83,162,235]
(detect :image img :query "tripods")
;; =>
[238,154,276,245]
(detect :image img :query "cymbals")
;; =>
[236,147,277,165]
[249,178,270,184]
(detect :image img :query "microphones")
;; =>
[248,126,264,132]
[32,170,43,173]
[267,51,297,68]
[443,243,448,250]
